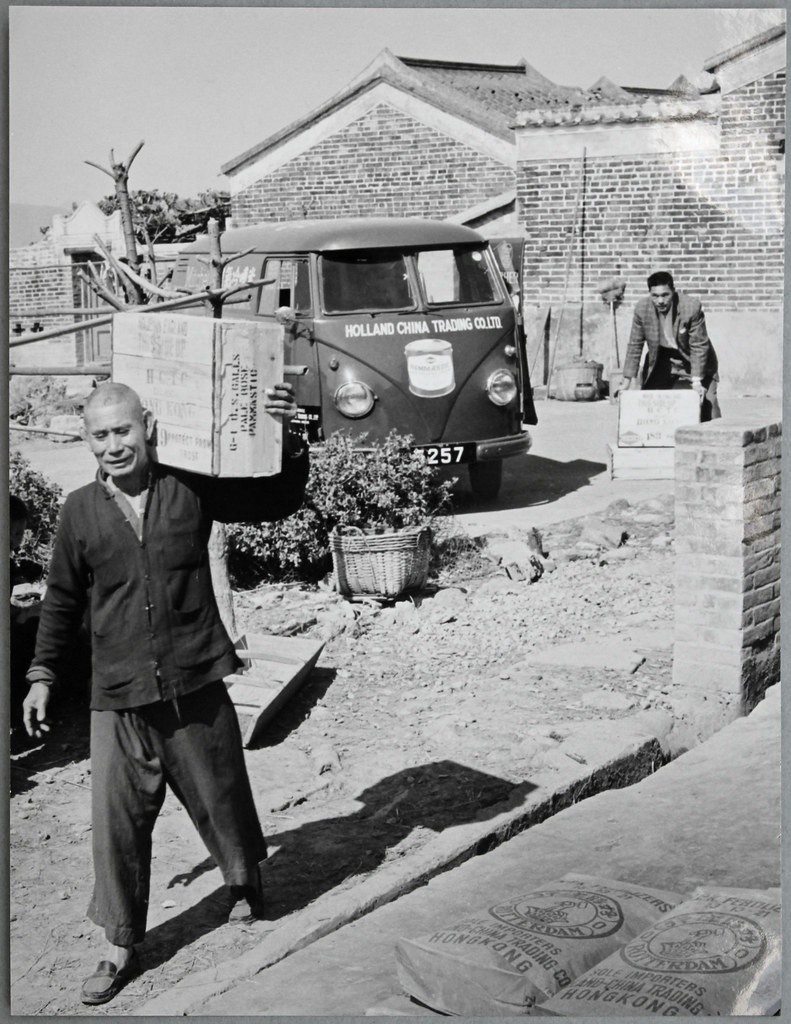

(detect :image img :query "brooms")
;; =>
[598,281,626,369]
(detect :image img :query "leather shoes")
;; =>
[228,892,261,925]
[81,944,140,1004]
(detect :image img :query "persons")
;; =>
[612,273,721,422]
[20,380,310,1007]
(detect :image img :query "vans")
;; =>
[181,220,539,499]
